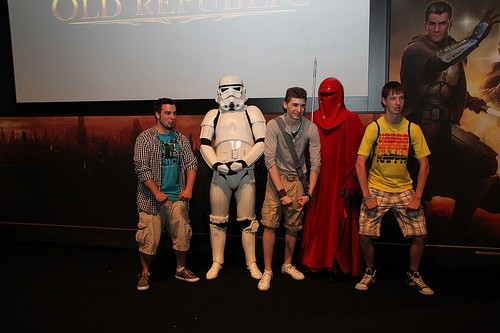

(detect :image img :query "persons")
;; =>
[134,98,201,289]
[300,77,366,276]
[258,87,321,291]
[201,77,267,280]
[355,82,434,295]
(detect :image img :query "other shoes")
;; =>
[206,262,222,279]
[247,262,262,279]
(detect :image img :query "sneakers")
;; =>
[175,267,200,282]
[354,268,377,290]
[405,271,434,295]
[258,269,273,290]
[281,264,305,279]
[137,273,151,290]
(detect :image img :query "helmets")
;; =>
[214,76,248,110]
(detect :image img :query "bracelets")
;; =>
[365,197,371,201]
[306,192,313,201]
[278,188,286,199]
